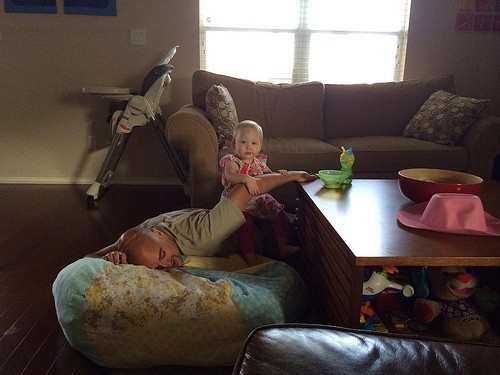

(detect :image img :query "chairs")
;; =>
[82,44,190,210]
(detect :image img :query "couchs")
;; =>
[165,69,500,209]
[232,322,500,375]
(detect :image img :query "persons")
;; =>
[220,120,299,266]
[83,171,318,269]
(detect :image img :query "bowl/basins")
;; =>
[319,170,352,189]
[398,169,483,204]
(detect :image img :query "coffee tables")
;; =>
[294,178,500,343]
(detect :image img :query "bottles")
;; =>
[340,146,355,185]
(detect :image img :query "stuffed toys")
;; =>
[413,267,490,339]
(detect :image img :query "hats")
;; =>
[398,192,500,236]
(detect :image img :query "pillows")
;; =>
[205,83,239,149]
[403,90,490,146]
